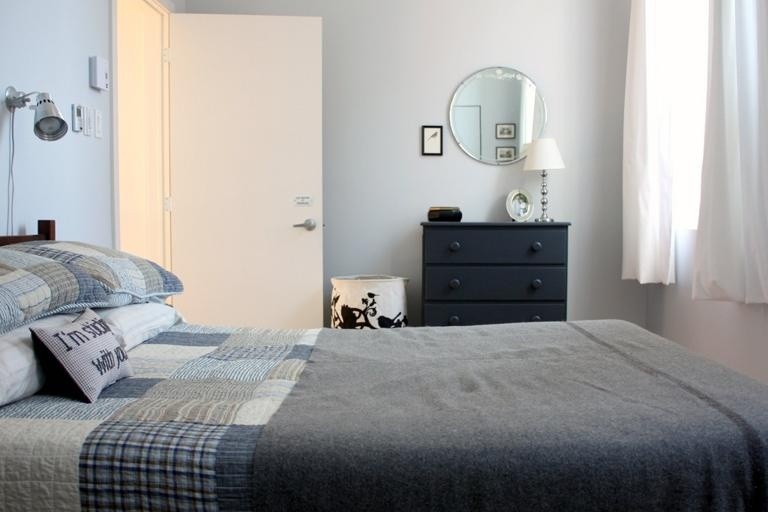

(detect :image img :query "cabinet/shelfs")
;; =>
[420,222,572,326]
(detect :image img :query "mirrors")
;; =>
[449,67,548,166]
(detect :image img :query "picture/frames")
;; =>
[422,126,443,156]
[505,189,534,223]
[453,105,516,163]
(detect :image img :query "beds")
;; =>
[0,219,767,511]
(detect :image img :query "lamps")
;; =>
[519,143,532,159]
[5,86,68,141]
[523,139,565,223]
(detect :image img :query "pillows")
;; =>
[1,239,184,405]
